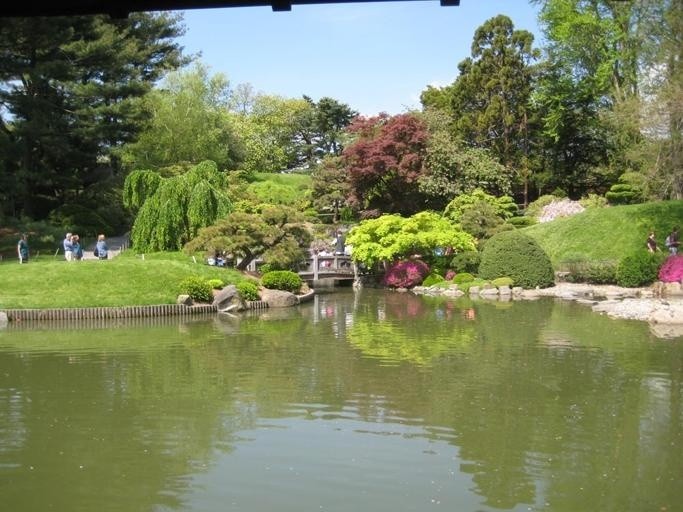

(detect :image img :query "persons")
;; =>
[63,232,75,262]
[669,226,681,255]
[646,231,664,253]
[73,234,83,260]
[96,234,109,260]
[208,250,234,267]
[17,234,29,264]
[318,231,352,268]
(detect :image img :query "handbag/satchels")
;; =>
[94,249,98,256]
